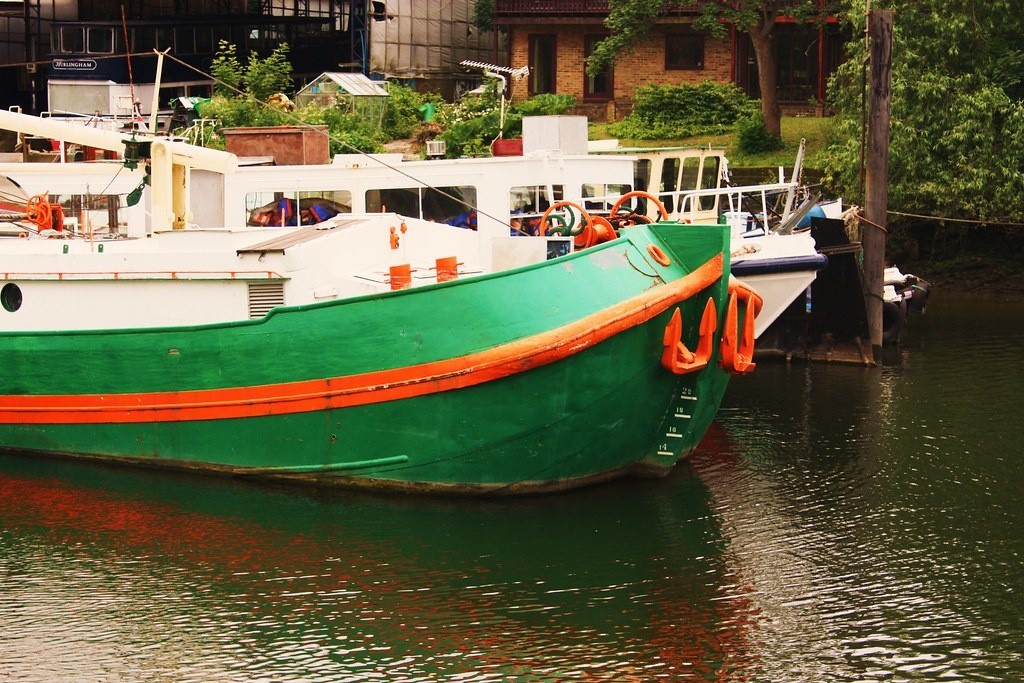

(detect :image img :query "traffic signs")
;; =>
[52,58,97,71]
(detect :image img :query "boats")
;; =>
[0,47,931,498]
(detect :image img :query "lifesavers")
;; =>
[864,301,904,340]
[910,281,932,314]
